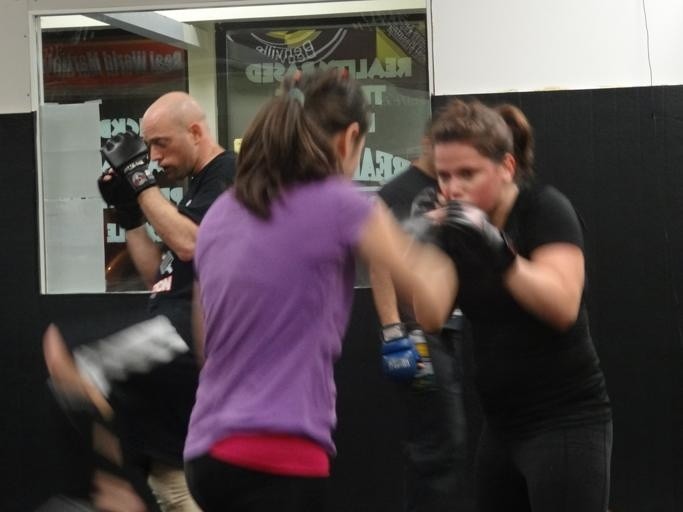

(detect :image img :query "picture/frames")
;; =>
[215,9,433,290]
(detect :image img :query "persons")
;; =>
[181,66,460,511]
[378,95,613,512]
[367,115,486,511]
[42,89,237,511]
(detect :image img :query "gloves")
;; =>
[446,203,515,273]
[380,324,421,380]
[103,131,157,193]
